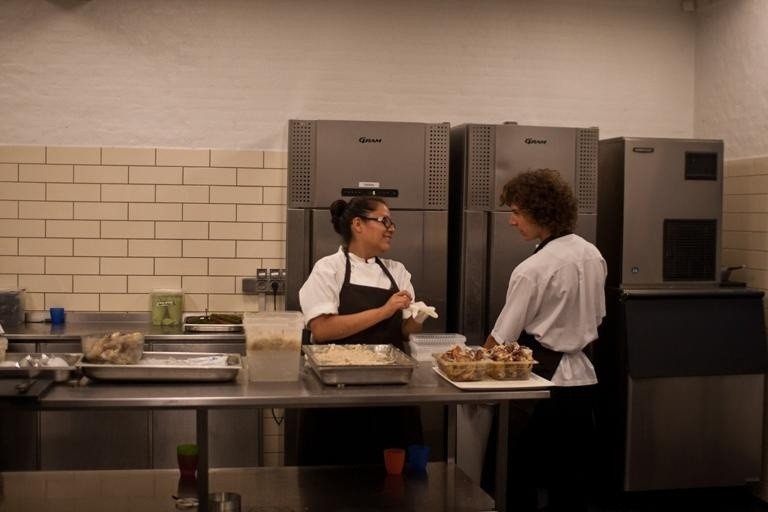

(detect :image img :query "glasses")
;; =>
[361,216,395,228]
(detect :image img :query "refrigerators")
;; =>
[455,122,602,339]
[281,117,454,330]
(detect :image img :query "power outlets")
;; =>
[256,279,285,293]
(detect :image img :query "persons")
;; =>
[481,169,608,512]
[295,195,423,465]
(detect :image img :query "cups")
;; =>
[383,447,429,474]
[178,444,199,476]
[49,307,65,322]
